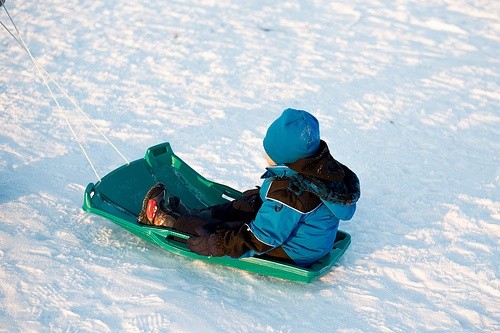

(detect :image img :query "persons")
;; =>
[137,108,360,268]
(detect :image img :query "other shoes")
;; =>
[137,183,180,228]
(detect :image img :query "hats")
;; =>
[263,107,320,164]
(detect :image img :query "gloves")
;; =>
[188,227,222,256]
[233,190,259,211]
